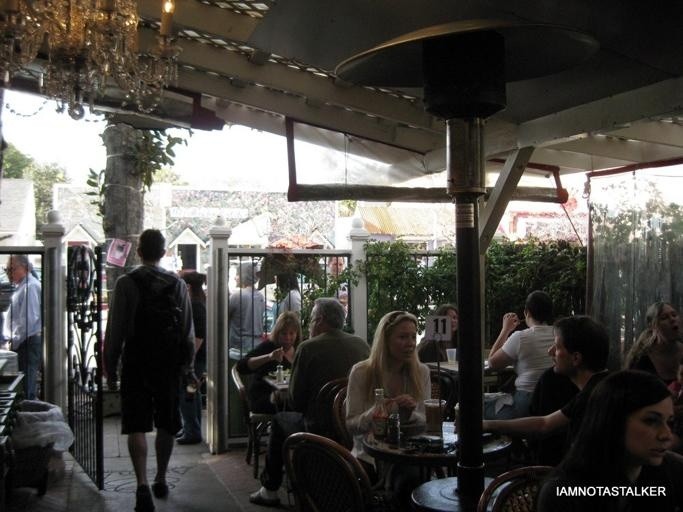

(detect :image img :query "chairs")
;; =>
[229,336,681,510]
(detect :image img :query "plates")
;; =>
[267,370,287,378]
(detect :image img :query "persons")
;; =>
[2,254,43,400]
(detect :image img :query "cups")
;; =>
[453,403,460,438]
[446,349,455,365]
[422,398,447,432]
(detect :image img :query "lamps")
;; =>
[0,1,184,125]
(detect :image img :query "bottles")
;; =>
[386,410,400,440]
[371,389,387,438]
[276,365,283,383]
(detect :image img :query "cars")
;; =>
[0,259,89,313]
[200,260,444,342]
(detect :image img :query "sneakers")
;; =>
[248,489,281,508]
[175,431,201,444]
